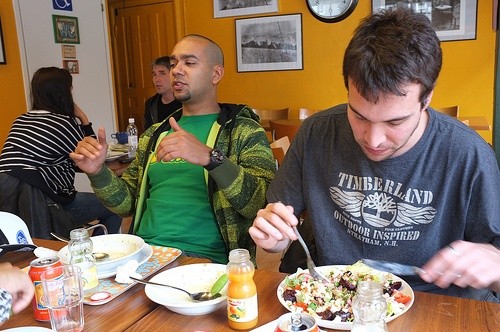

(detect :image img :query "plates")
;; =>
[277,265,414,330]
[105,150,127,161]
[110,143,128,152]
[99,243,153,280]
[0,326,57,332]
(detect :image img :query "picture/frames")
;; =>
[0,15,7,65]
[52,15,80,45]
[234,13,305,74]
[372,0,478,42]
[213,0,281,20]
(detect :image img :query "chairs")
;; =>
[251,106,290,144]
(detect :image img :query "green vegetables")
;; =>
[285,271,395,313]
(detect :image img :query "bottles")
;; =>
[68,228,99,297]
[226,249,258,330]
[351,280,388,332]
[127,118,138,160]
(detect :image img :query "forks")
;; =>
[292,226,329,284]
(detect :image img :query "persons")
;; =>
[248,8,500,303]
[69,34,277,269]
[0,263,36,327]
[0,67,122,237]
[144,55,183,128]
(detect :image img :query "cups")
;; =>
[40,266,84,332]
[299,108,308,120]
[111,132,128,144]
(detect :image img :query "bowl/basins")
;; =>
[57,234,145,274]
[144,263,230,316]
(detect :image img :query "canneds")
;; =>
[274,311,320,332]
[27,256,68,322]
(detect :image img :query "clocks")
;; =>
[305,0,359,24]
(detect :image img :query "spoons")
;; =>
[50,232,107,258]
[129,276,221,302]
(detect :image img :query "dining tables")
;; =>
[0,238,500,332]
